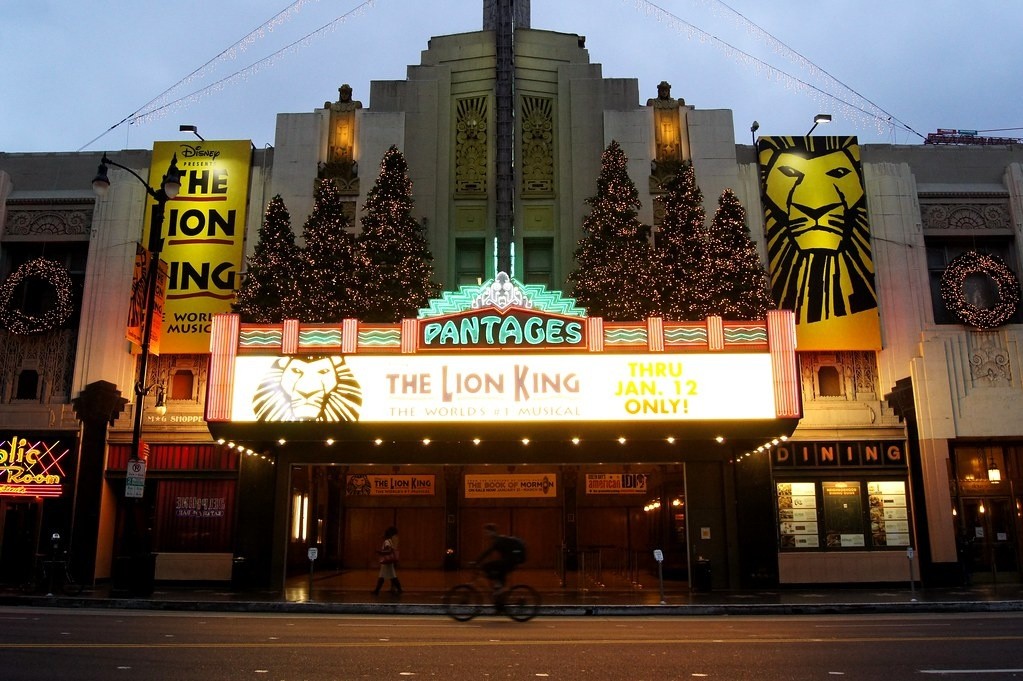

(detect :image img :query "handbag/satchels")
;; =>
[379,540,399,564]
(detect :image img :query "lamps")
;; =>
[179,125,205,141]
[987,447,1001,484]
[806,115,832,136]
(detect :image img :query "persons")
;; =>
[472,523,516,616]
[372,526,404,596]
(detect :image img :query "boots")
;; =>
[391,577,403,596]
[371,577,384,596]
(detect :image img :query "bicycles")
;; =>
[443,562,544,622]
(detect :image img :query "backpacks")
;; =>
[497,536,526,564]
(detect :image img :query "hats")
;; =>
[484,524,497,531]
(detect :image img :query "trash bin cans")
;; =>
[693,559,713,593]
[42,560,70,595]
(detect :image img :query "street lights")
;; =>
[91,150,183,613]
